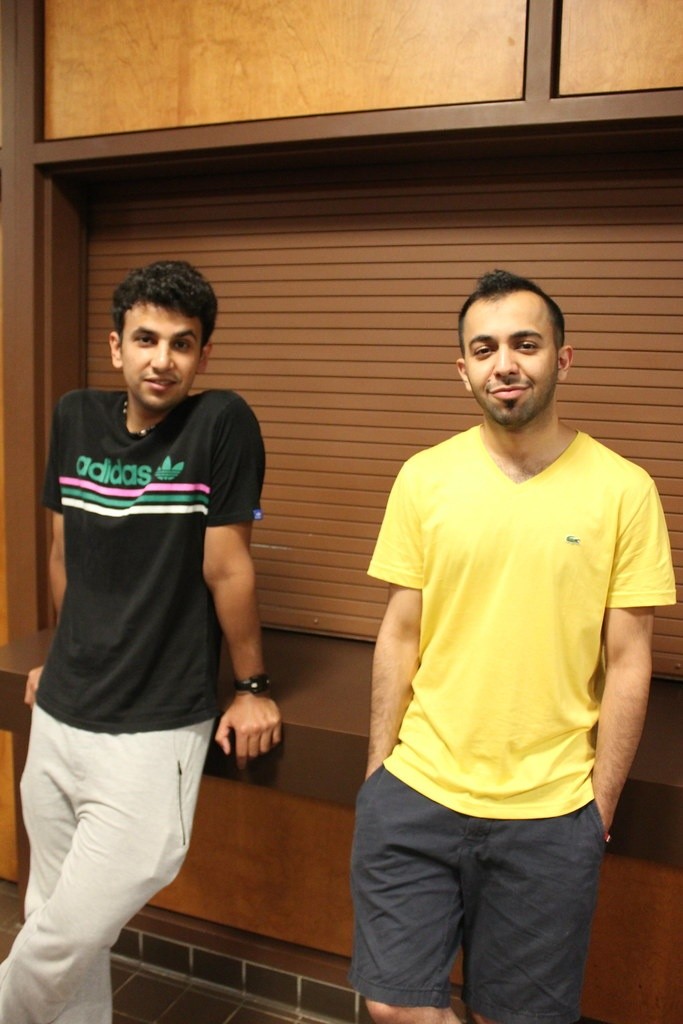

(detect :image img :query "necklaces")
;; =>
[123,402,155,437]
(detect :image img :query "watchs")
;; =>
[233,672,269,694]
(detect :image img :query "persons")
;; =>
[348,273,659,1024]
[0,261,282,1024]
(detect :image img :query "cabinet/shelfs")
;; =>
[2,0,683,192]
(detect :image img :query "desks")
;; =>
[2,622,681,875]
